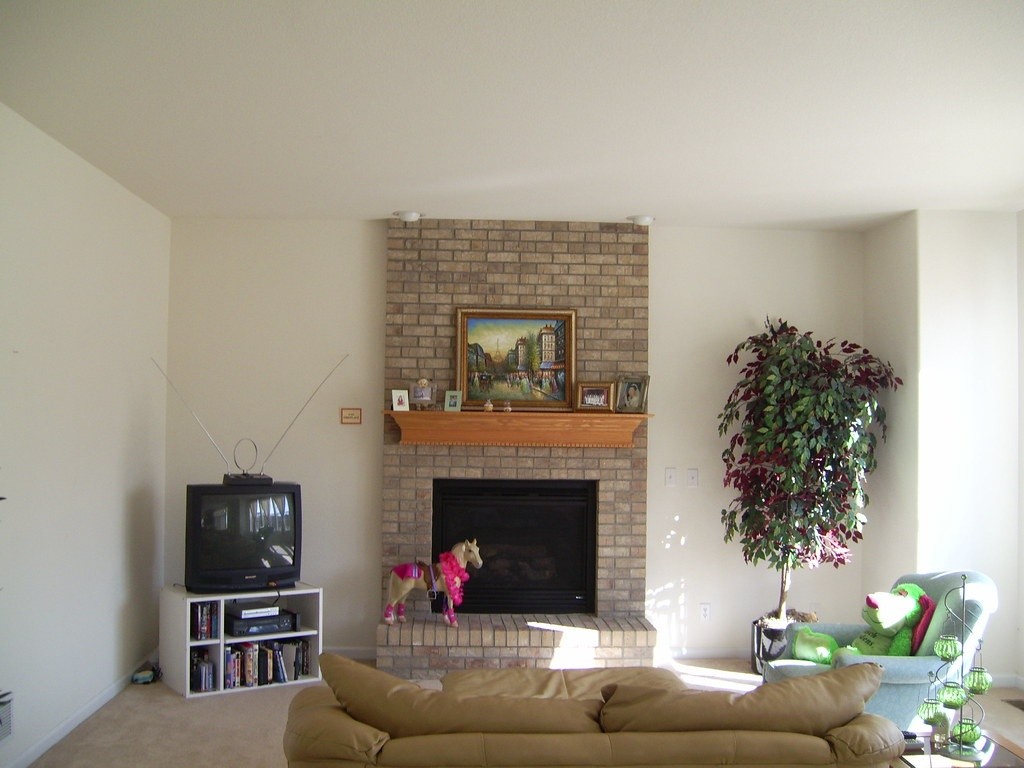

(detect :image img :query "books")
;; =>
[224,638,311,689]
[193,602,218,640]
[192,650,216,692]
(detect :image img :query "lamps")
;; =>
[627,215,655,227]
[394,209,426,223]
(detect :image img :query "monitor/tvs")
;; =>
[184,481,302,594]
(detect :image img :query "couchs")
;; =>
[280,652,908,768]
[761,571,996,735]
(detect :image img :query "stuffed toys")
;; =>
[793,583,936,666]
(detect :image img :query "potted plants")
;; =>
[717,319,903,672]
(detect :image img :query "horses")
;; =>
[383,538,483,627]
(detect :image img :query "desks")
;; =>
[887,730,1024,768]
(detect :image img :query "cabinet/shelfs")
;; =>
[160,584,325,697]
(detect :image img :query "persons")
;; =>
[585,393,605,405]
[624,383,641,407]
[449,395,457,407]
[398,395,404,405]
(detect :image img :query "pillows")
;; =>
[599,662,887,735]
[316,652,603,736]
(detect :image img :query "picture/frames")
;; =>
[392,390,409,411]
[453,305,577,412]
[616,374,650,413]
[409,383,437,405]
[445,391,461,412]
[576,380,617,414]
[340,408,361,424]
